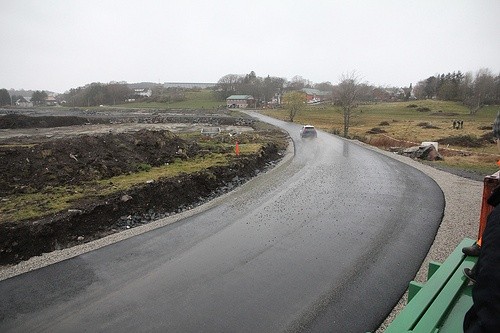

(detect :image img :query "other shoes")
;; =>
[463,268,479,284]
[462,244,481,257]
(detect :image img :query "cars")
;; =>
[299,125,317,138]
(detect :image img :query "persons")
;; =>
[453,121,456,129]
[457,120,460,128]
[462,186,500,333]
[460,120,463,128]
